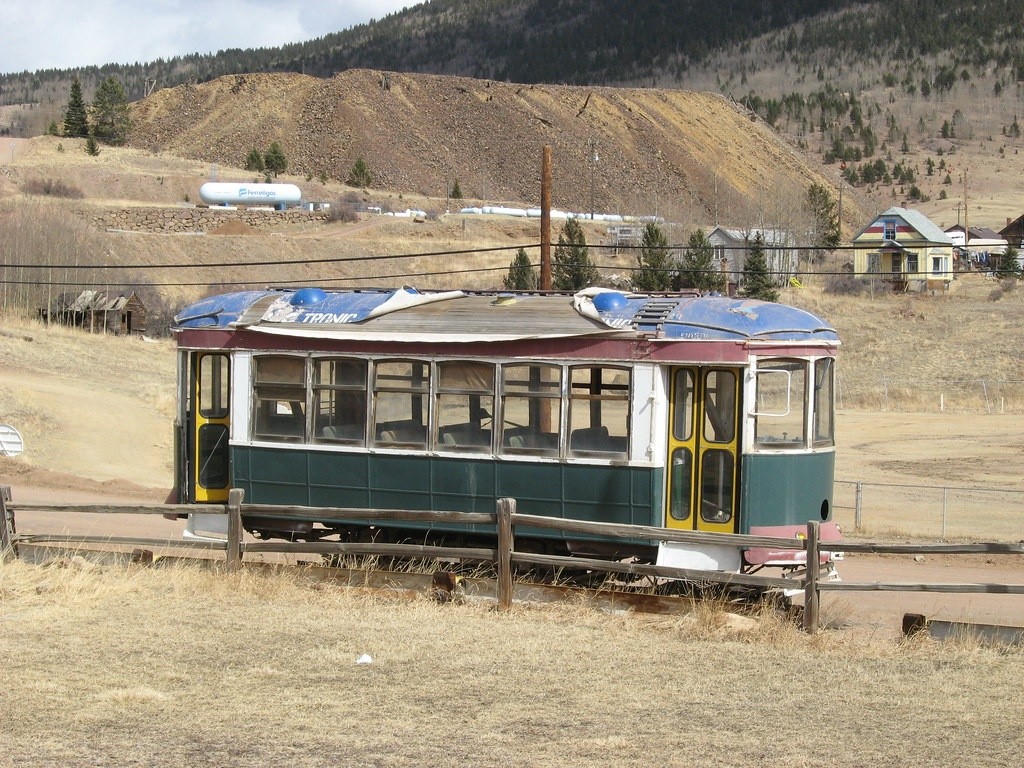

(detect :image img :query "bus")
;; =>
[162,287,847,606]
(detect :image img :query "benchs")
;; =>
[323,418,611,451]
